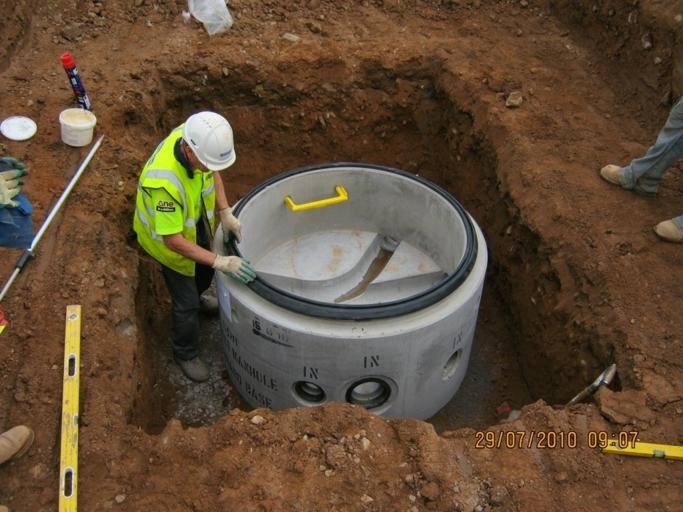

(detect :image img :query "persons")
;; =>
[1,424,37,511]
[600,94,683,242]
[132,108,256,381]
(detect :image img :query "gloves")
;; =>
[0,156,30,210]
[220,207,242,244]
[212,255,256,285]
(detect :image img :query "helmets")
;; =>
[182,110,237,171]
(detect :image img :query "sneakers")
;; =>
[174,354,210,382]
[601,164,654,196]
[653,219,682,242]
[200,295,220,314]
[1,425,35,467]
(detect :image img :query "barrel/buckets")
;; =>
[59,109,97,146]
